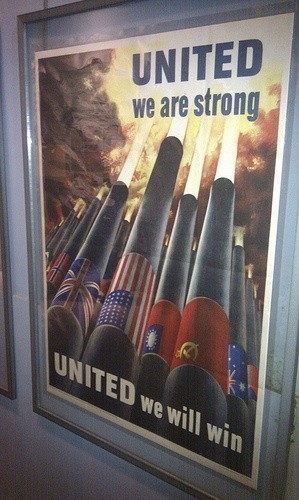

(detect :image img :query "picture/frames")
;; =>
[15,0,298,500]
